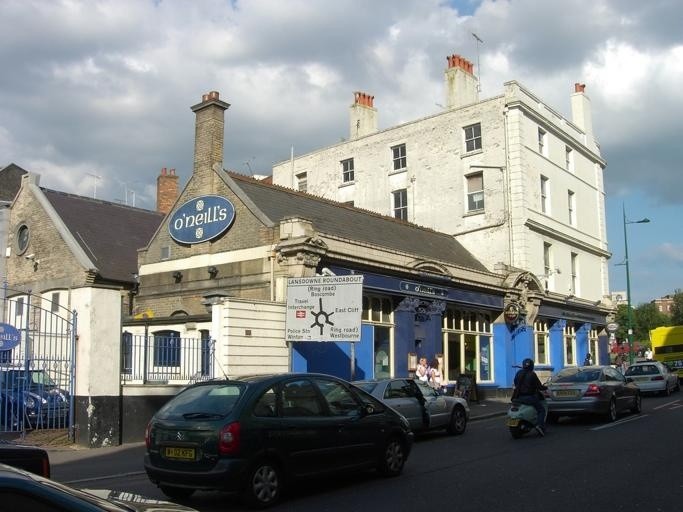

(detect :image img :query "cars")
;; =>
[0,458,196,512]
[0,362,67,431]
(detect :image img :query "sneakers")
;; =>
[535,424,545,437]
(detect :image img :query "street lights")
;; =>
[612,199,650,361]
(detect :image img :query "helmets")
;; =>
[522,358,534,371]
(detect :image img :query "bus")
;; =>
[645,321,683,377]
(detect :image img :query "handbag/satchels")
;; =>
[415,370,425,377]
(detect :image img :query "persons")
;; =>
[614,346,652,379]
[584,353,594,366]
[416,356,430,384]
[511,358,549,437]
[428,359,444,396]
